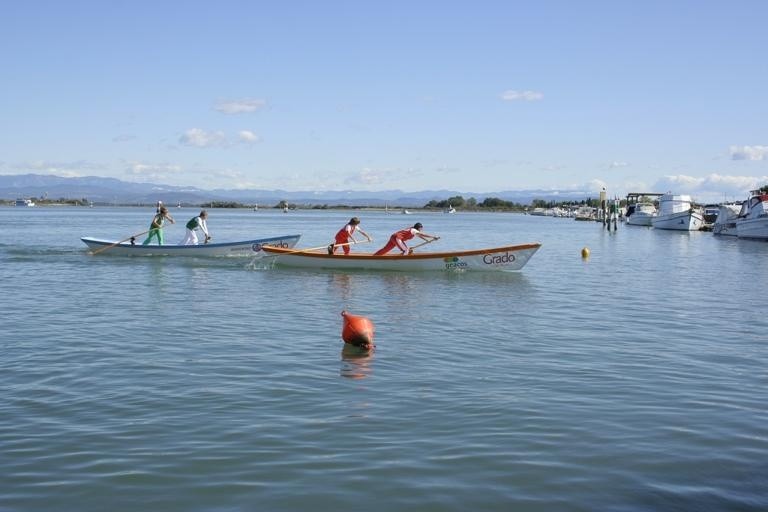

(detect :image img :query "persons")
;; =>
[371,221,441,256]
[328,215,371,254]
[177,209,210,247]
[142,206,175,245]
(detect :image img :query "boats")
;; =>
[262,241,545,272]
[77,233,303,265]
[532,191,768,240]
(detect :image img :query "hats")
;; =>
[349,217,360,225]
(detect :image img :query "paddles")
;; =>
[88,222,172,255]
[264,240,367,258]
